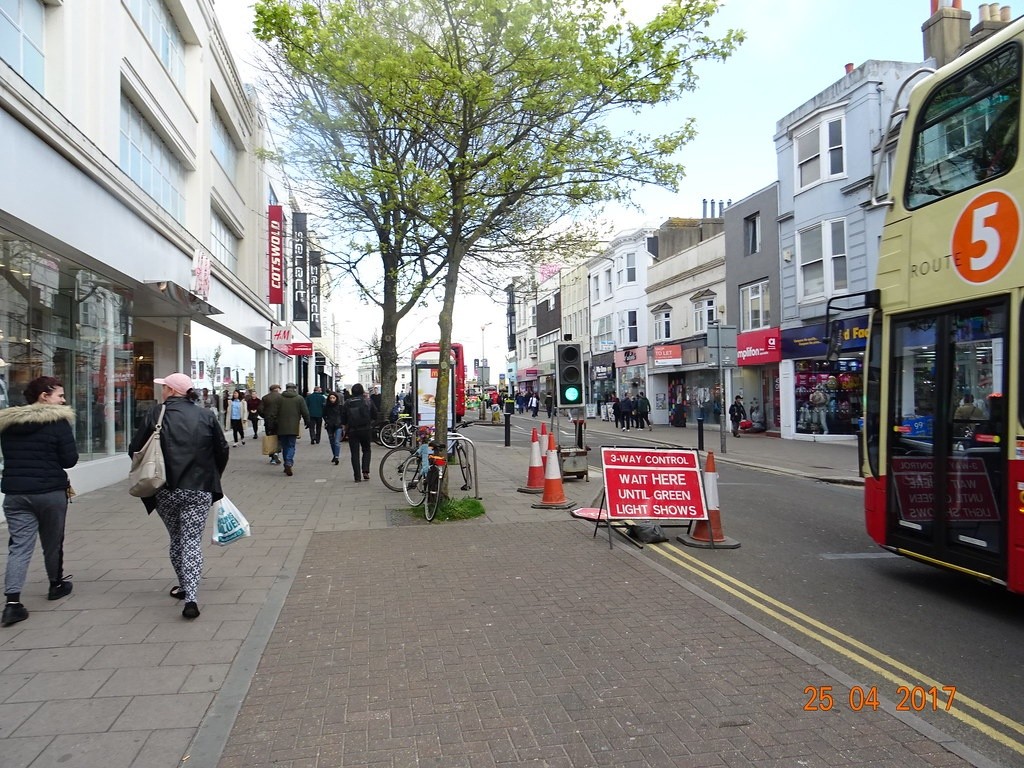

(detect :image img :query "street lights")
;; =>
[481,324,486,408]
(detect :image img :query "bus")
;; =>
[820,12,1023,594]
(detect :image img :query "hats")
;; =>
[154,373,193,395]
[735,395,743,399]
[286,383,297,389]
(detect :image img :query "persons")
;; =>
[729,396,746,438]
[0,359,9,480]
[604,389,653,432]
[186,387,252,431]
[479,388,569,418]
[809,384,829,434]
[696,397,722,425]
[0,376,79,623]
[128,373,229,617]
[232,383,413,482]
[952,390,989,421]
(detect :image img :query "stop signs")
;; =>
[570,507,611,520]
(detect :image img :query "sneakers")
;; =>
[48,575,73,599]
[1,604,29,624]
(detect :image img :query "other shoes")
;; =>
[233,443,239,447]
[311,439,315,444]
[616,424,652,432]
[363,472,370,479]
[284,464,293,476]
[317,440,320,444]
[170,586,187,599]
[355,478,360,483]
[734,433,740,438]
[274,453,281,463]
[270,460,276,464]
[253,434,257,439]
[241,438,245,445]
[183,601,201,620]
[332,456,339,465]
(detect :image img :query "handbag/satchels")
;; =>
[262,435,281,455]
[213,494,252,547]
[128,404,167,497]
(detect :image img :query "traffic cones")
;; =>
[677,450,741,550]
[540,423,549,473]
[516,428,545,494]
[531,432,577,509]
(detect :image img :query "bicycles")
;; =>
[378,418,478,492]
[371,414,413,449]
[402,425,446,522]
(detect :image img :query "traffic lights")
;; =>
[555,340,586,408]
